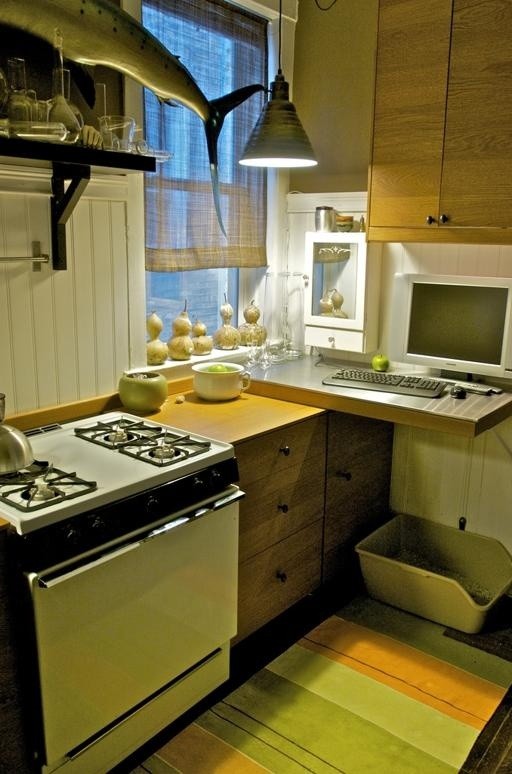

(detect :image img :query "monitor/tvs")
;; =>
[389,273,512,393]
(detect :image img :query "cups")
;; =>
[315,206,335,231]
[192,362,251,400]
[95,115,136,150]
[258,327,270,369]
[244,324,258,367]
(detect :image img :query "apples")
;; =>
[373,355,391,371]
[205,363,230,373]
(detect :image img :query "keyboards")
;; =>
[323,369,447,398]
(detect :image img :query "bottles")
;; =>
[1,120,67,142]
[264,270,304,360]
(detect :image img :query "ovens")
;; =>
[23,484,244,764]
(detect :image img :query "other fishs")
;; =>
[0,0,273,240]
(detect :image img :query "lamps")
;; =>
[238,0,318,168]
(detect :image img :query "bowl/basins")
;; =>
[336,215,353,232]
[118,372,168,414]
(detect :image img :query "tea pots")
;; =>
[0,392,35,473]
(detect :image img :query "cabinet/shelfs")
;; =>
[304,231,367,356]
[369,0,511,231]
[235,413,328,654]
[323,414,395,584]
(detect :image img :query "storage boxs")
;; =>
[354,513,512,635]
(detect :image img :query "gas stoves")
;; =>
[0,412,241,563]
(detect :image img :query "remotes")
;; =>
[455,383,492,395]
